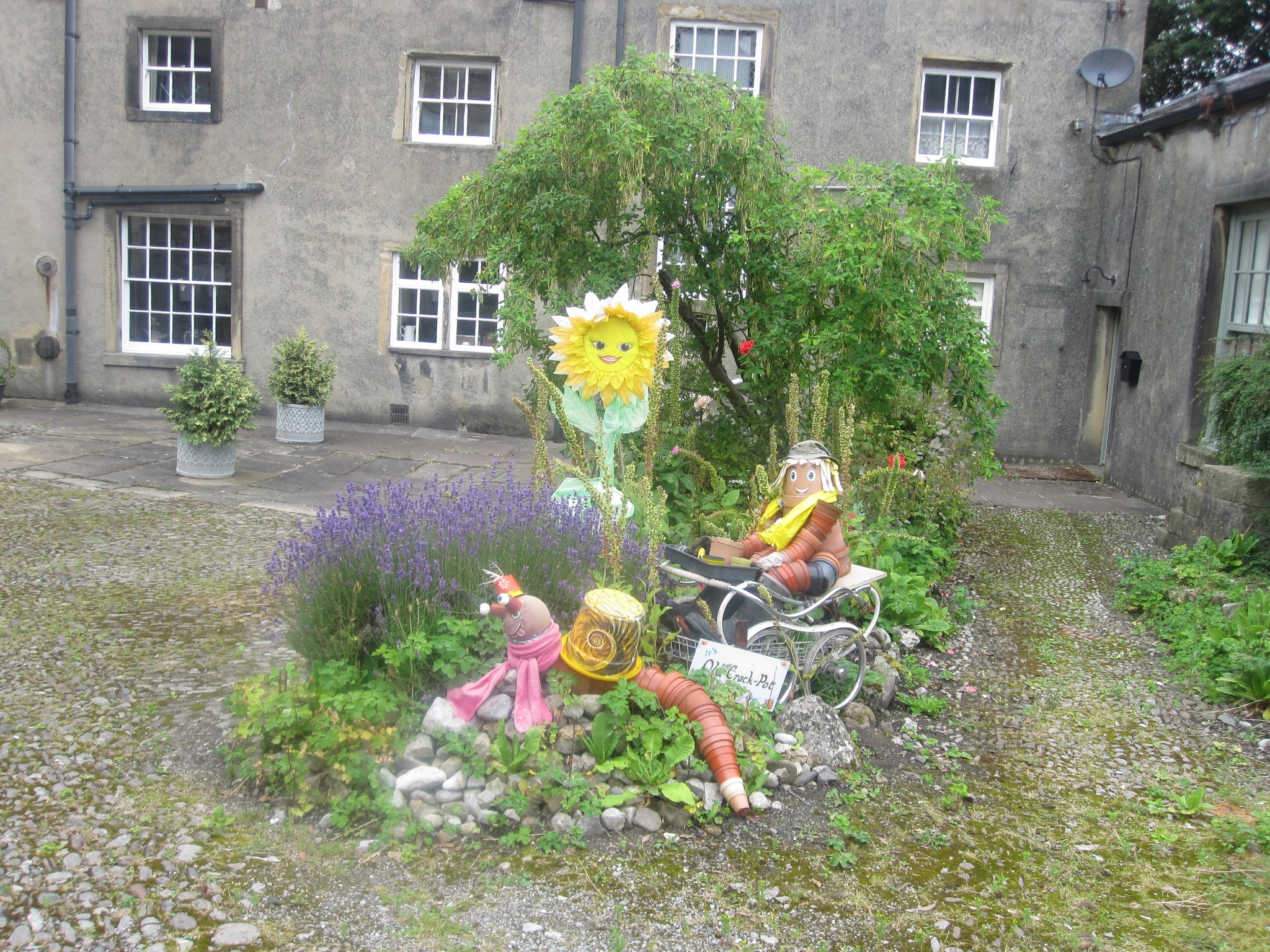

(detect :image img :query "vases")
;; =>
[731,557,753,567]
[702,555,725,567]
[709,536,744,565]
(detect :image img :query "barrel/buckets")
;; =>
[559,588,645,681]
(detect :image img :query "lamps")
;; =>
[1080,266,1115,289]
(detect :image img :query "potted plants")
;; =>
[266,327,338,445]
[155,328,263,481]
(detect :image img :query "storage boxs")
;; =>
[665,544,760,582]
